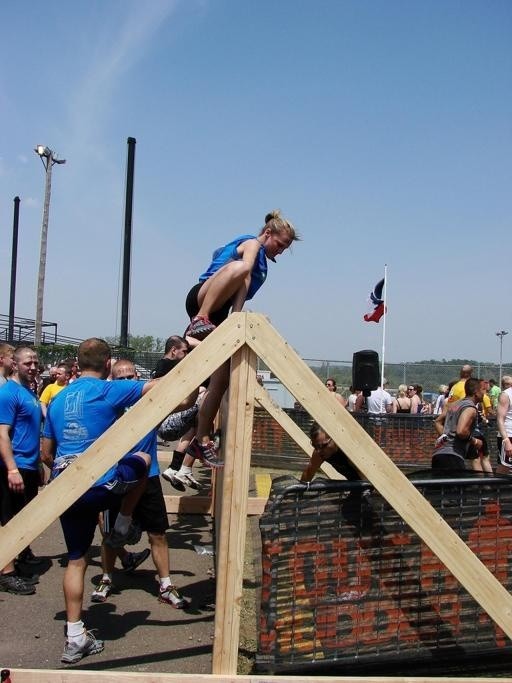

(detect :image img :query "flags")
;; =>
[362,278,387,324]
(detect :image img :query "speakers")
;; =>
[353,349,380,391]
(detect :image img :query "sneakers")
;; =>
[211,429,221,450]
[121,547,151,571]
[162,466,186,492]
[187,438,225,470]
[186,315,217,337]
[158,584,188,609]
[103,522,143,549]
[61,627,105,663]
[174,468,204,490]
[91,579,113,601]
[0,551,40,594]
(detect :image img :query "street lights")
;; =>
[31,142,68,346]
[495,329,508,387]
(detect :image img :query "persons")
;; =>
[185,208,304,470]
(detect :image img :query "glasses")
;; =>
[312,437,331,448]
[408,389,414,391]
[114,376,135,381]
